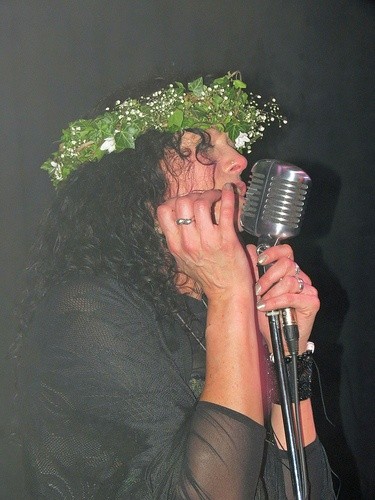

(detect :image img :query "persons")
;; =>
[2,72,342,500]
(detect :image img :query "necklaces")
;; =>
[166,298,208,354]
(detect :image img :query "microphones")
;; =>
[238,156,312,242]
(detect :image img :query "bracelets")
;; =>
[264,341,316,364]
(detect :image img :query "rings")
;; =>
[175,216,197,225]
[296,278,304,294]
[291,263,301,277]
